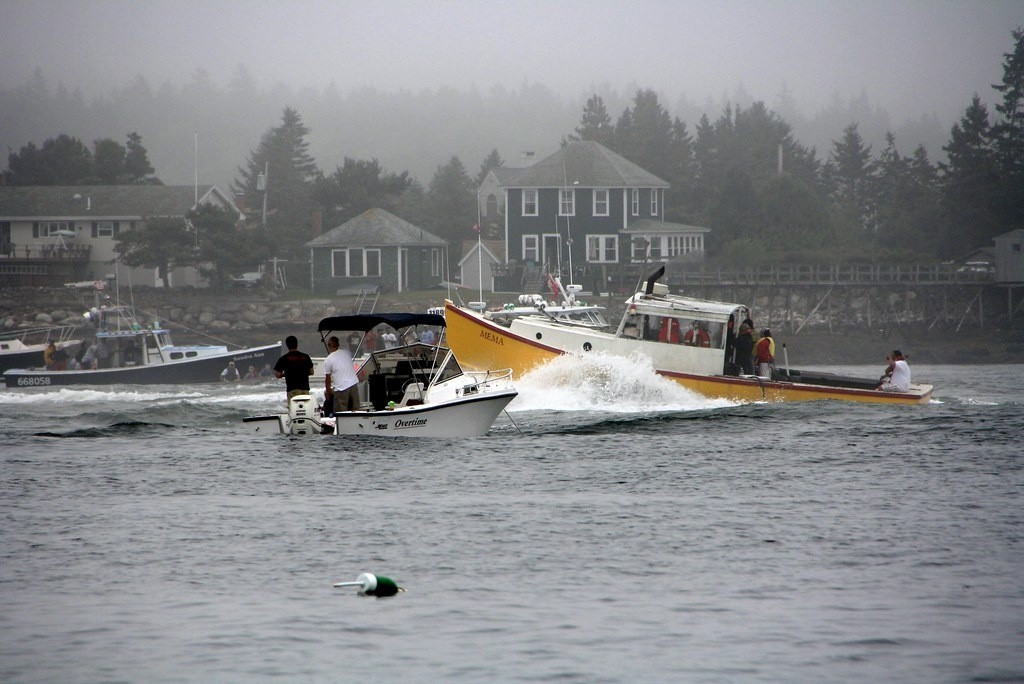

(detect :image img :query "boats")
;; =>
[0,325,84,378]
[307,274,613,390]
[442,265,935,405]
[2,280,283,389]
[241,312,521,439]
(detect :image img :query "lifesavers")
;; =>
[658,325,679,344]
[683,328,711,348]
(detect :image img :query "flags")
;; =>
[546,275,558,301]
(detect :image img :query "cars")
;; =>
[232,272,273,288]
[956,261,996,277]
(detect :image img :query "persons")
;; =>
[123,340,140,367]
[220,361,241,383]
[879,351,910,393]
[273,336,314,405]
[42,338,113,369]
[752,328,775,379]
[347,325,438,357]
[243,364,264,381]
[715,316,756,375]
[324,336,361,411]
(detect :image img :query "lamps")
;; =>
[105,273,116,280]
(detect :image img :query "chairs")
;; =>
[385,375,410,404]
